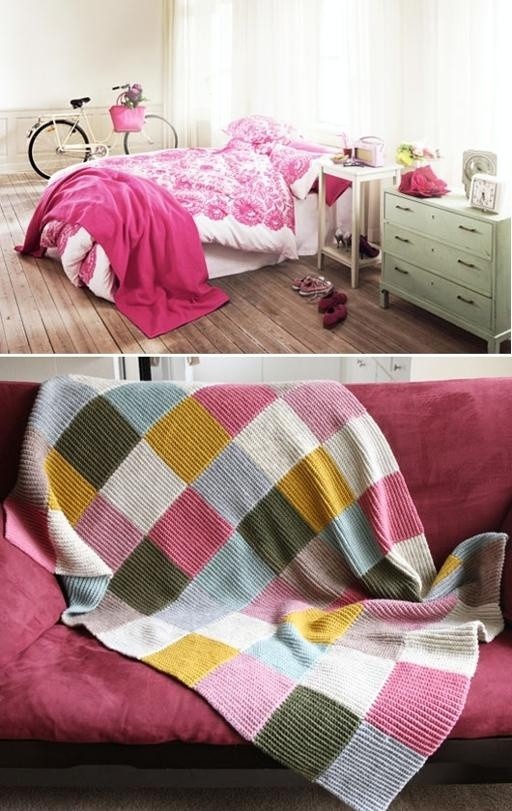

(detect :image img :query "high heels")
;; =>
[334,228,380,260]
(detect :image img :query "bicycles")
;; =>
[27,84,178,179]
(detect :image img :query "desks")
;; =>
[316,158,403,289]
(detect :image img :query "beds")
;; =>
[49,141,351,298]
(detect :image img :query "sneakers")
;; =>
[291,275,348,330]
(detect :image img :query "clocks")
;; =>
[460,150,502,215]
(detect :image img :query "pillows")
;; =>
[222,113,354,206]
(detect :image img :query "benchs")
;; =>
[1,376,511,789]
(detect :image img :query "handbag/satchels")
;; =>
[109,91,146,133]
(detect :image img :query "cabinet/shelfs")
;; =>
[378,186,511,354]
